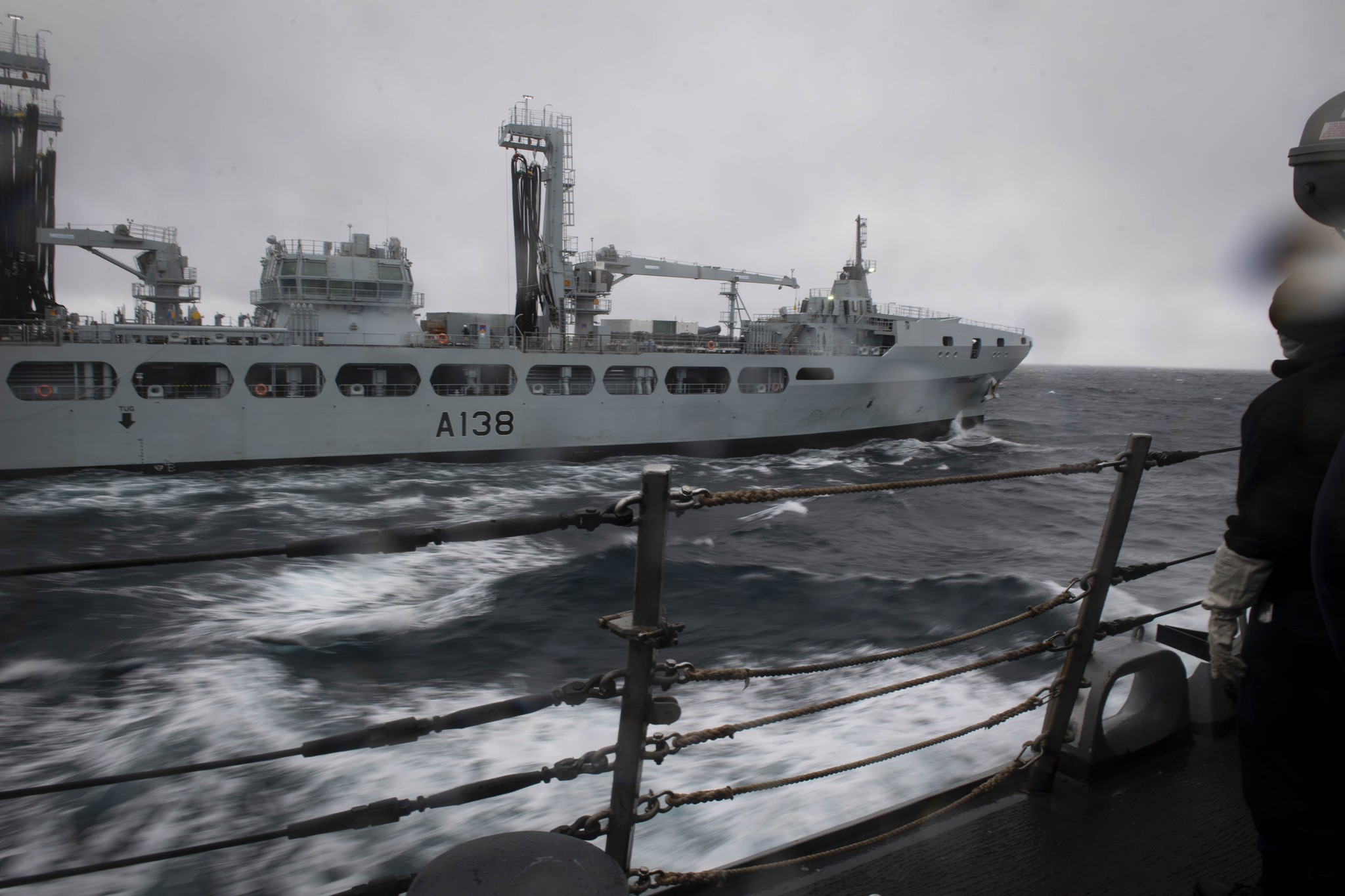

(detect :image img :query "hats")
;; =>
[1269,276,1345,343]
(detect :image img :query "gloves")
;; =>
[1207,545,1272,683]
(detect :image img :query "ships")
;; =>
[0,14,1032,486]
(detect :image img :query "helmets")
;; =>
[740,335,744,337]
[463,324,468,327]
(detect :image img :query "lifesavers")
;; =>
[36,385,52,398]
[437,333,448,344]
[707,340,715,350]
[771,383,779,392]
[254,383,268,396]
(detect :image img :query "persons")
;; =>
[738,335,747,354]
[461,325,470,345]
[1199,273,1345,896]
